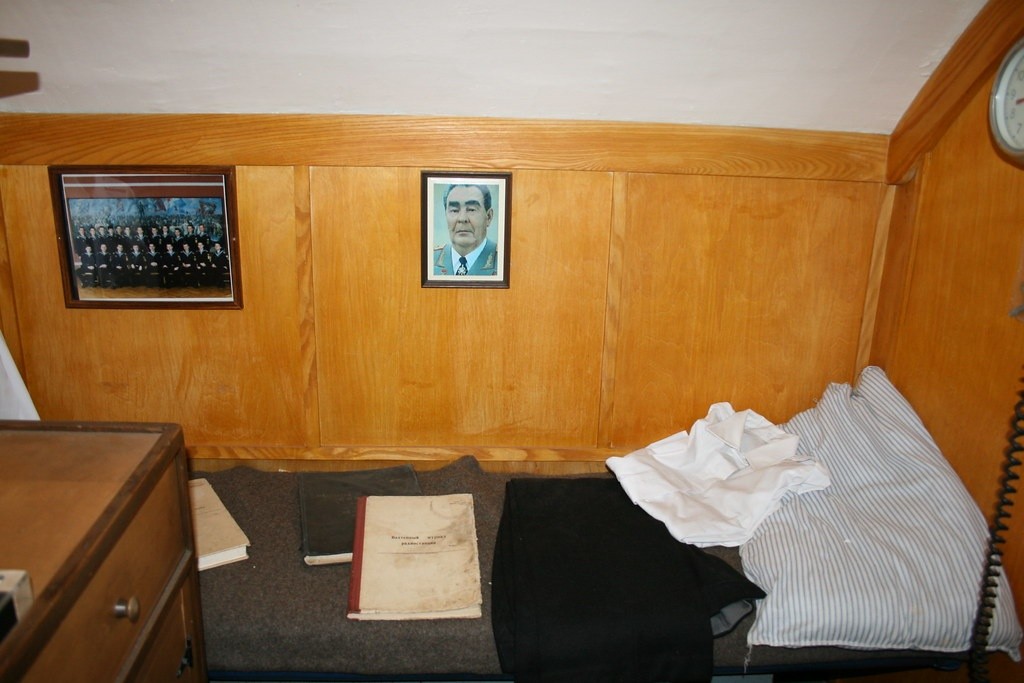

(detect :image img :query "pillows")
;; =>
[739,365,1023,663]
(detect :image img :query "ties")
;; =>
[455,257,468,276]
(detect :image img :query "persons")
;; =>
[433,184,498,275]
[75,224,228,288]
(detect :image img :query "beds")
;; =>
[192,455,972,682]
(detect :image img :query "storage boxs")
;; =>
[1,569,33,641]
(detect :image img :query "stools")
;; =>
[84,273,230,288]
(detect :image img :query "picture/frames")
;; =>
[47,165,244,310]
[422,170,512,289]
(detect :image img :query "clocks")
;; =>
[988,38,1023,159]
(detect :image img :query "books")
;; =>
[189,478,251,572]
[299,463,424,568]
[346,493,482,619]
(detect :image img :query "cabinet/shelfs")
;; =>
[0,419,209,683]
